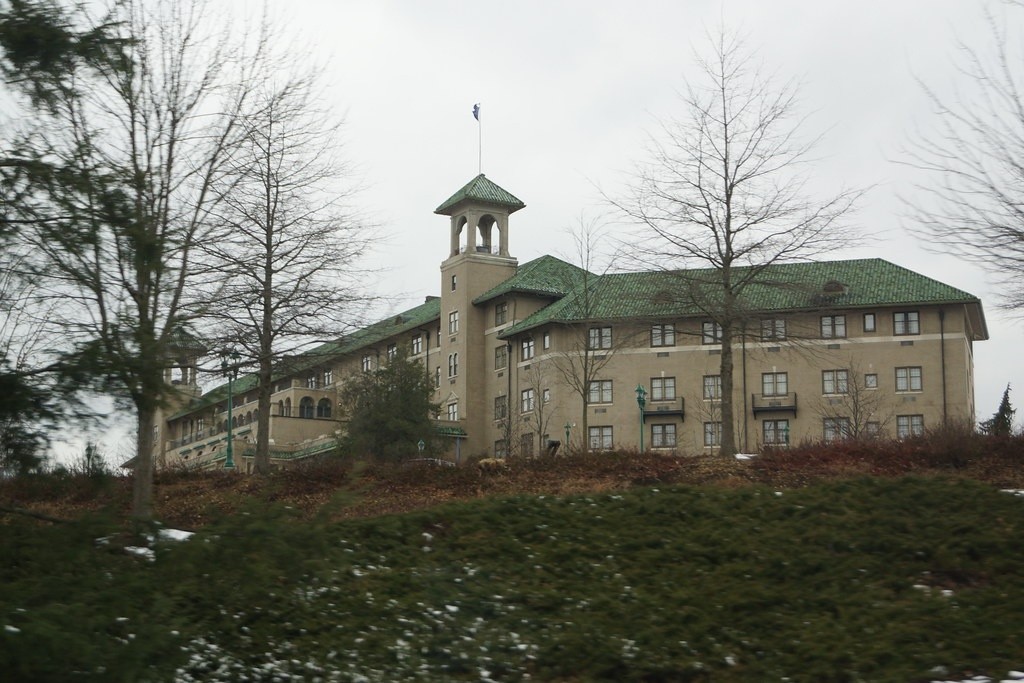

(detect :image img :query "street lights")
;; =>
[219,346,242,471]
[85,441,93,478]
[564,421,571,454]
[634,383,648,454]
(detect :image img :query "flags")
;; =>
[472,105,481,121]
